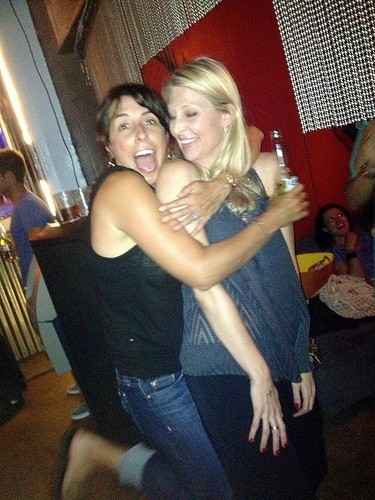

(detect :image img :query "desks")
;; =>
[31,219,125,437]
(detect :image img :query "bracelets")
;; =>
[346,252,358,259]
[253,219,271,241]
[223,169,237,186]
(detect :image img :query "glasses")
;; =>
[327,213,343,224]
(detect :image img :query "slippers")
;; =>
[53,425,80,500]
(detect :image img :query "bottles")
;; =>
[269,128,298,197]
[0,223,14,261]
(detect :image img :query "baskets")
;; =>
[296,252,334,299]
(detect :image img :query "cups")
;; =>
[54,190,81,225]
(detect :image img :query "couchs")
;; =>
[297,233,375,419]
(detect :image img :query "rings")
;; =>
[190,212,199,221]
[269,425,279,430]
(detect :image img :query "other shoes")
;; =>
[0,396,25,424]
[71,403,92,420]
[67,384,81,394]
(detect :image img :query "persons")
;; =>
[315,203,375,280]
[56,84,307,500]
[157,57,317,500]
[0,151,90,420]
